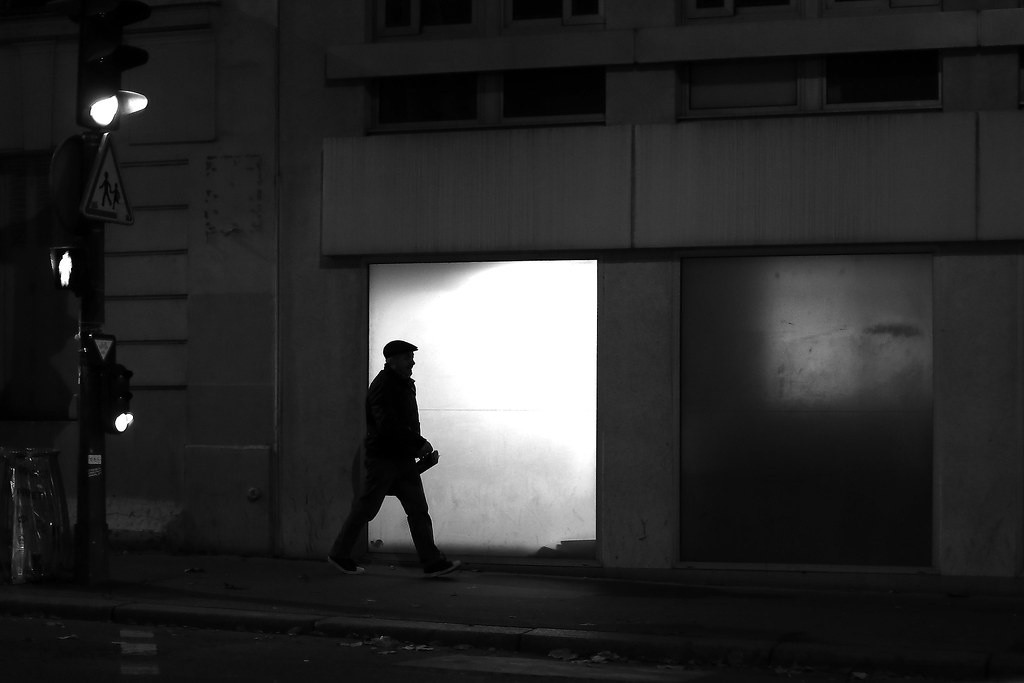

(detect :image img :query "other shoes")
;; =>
[424,560,461,578]
[328,554,364,574]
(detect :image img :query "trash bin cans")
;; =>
[0,448,75,586]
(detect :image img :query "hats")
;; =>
[383,341,418,358]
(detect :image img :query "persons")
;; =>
[324,340,466,581]
